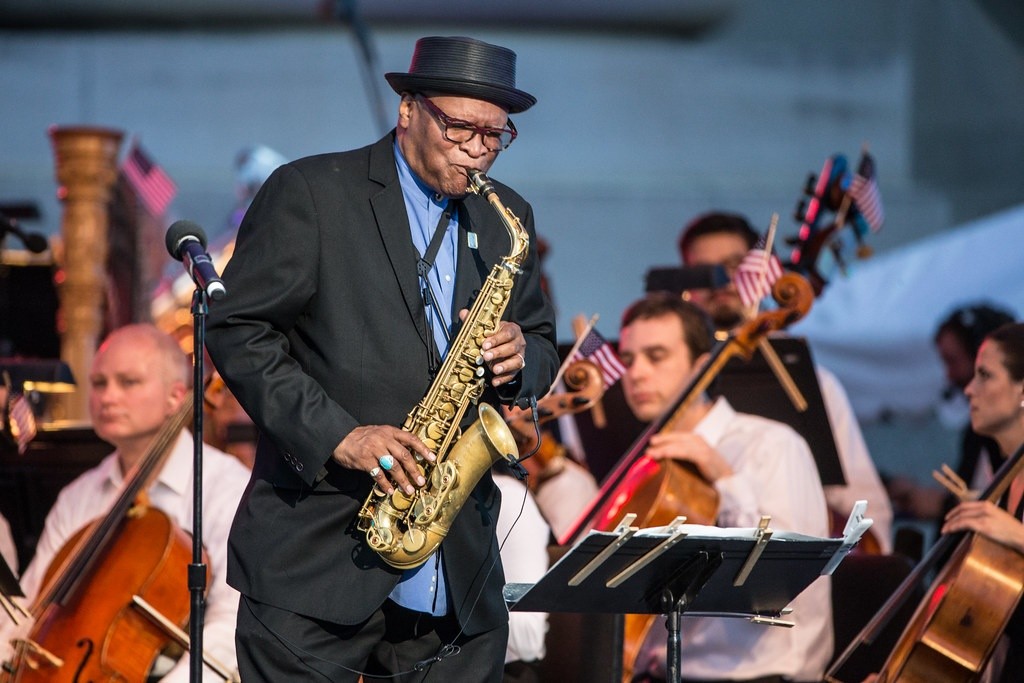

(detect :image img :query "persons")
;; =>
[0,212,1024,683]
[201,33,561,682]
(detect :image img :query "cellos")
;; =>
[824,441,1024,683]
[0,365,228,683]
[556,270,812,683]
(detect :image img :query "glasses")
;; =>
[420,93,518,151]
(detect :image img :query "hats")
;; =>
[384,37,538,115]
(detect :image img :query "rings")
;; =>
[379,454,393,470]
[516,353,525,370]
[370,467,380,476]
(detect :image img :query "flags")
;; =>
[850,152,886,235]
[736,227,784,307]
[123,145,175,211]
[570,326,626,389]
[9,390,43,452]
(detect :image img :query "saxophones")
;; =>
[357,165,530,569]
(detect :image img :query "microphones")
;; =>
[517,395,539,422]
[165,220,226,302]
